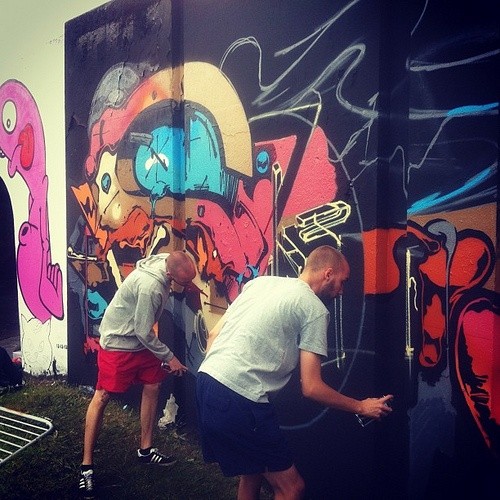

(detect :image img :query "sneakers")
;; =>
[78,462,95,499]
[137,447,178,466]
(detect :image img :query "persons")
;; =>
[194,246,394,500]
[78,250,196,500]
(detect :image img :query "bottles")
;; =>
[356,394,392,427]
[161,362,188,376]
